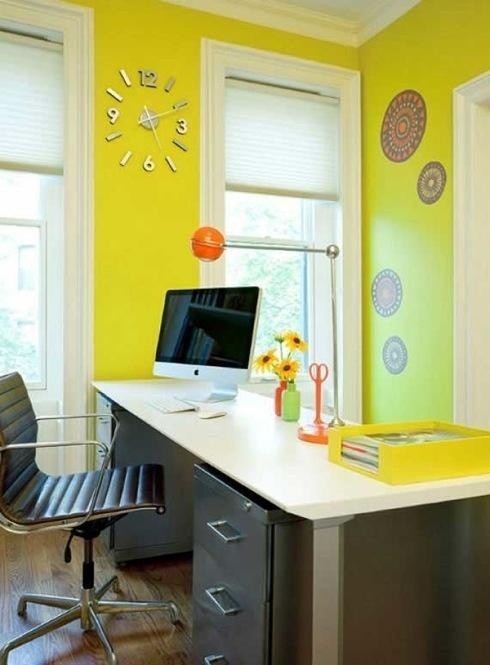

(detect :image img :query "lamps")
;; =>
[192,228,350,431]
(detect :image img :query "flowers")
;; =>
[258,332,310,384]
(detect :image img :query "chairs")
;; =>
[0,372,184,665]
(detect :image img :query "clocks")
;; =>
[102,64,196,177]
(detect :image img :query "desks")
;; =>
[95,376,488,664]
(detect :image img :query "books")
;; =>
[340,431,464,476]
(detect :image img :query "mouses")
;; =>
[197,408,226,419]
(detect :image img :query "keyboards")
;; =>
[148,397,195,414]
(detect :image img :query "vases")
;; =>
[272,383,302,423]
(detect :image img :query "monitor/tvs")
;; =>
[152,285,262,403]
